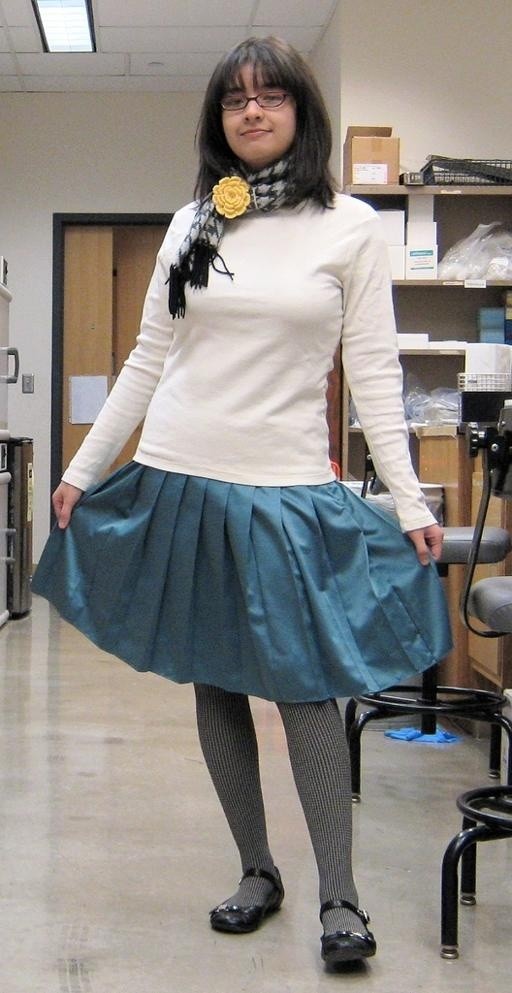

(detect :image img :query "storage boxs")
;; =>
[342,125,401,186]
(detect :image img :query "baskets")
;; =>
[458,372,512,393]
[425,157,512,185]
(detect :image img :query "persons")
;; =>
[47,32,446,967]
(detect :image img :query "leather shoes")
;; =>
[319,897,376,962]
[210,865,285,932]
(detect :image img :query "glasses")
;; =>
[218,90,294,111]
